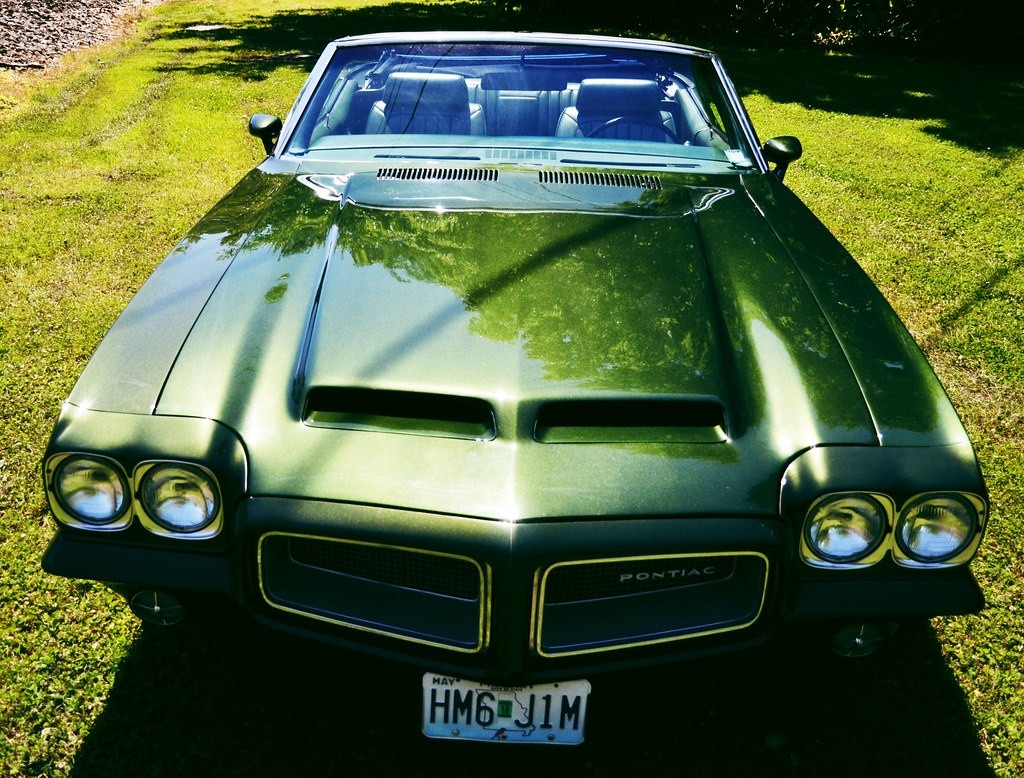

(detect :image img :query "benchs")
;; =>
[345,77,578,137]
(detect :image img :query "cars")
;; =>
[41,29,990,746]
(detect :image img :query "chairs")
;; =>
[555,77,683,144]
[362,71,486,134]
